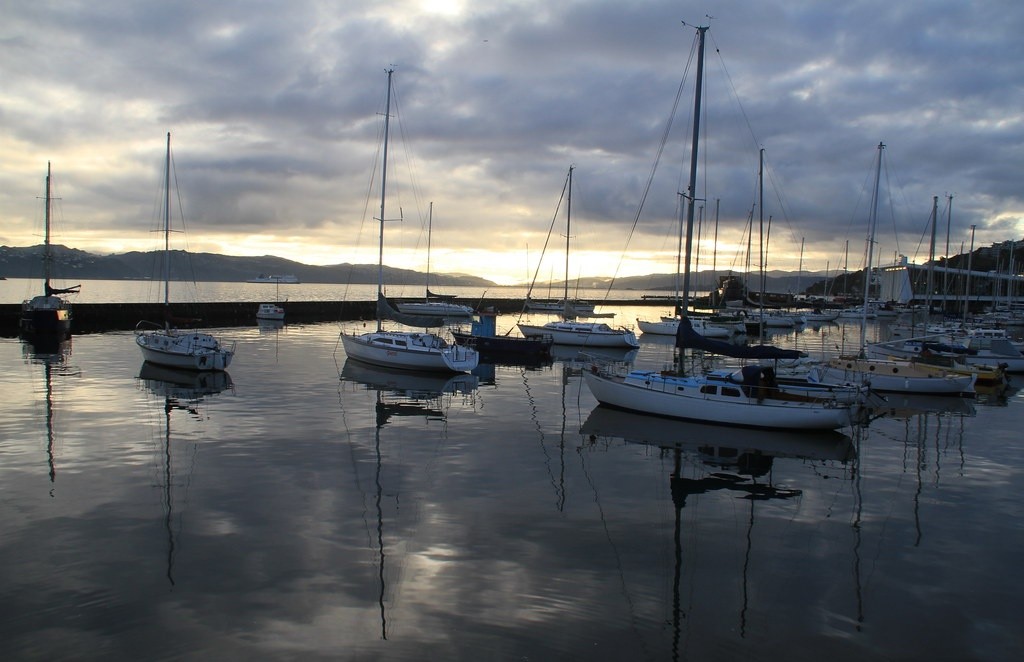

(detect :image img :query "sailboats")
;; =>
[520,346,653,511]
[337,356,478,641]
[875,391,977,547]
[395,201,475,317]
[133,132,237,370]
[583,13,1024,426]
[338,63,480,371]
[516,161,638,345]
[22,340,74,481]
[18,160,81,345]
[580,406,860,662]
[133,359,237,585]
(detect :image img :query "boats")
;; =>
[247,273,300,284]
[256,278,285,319]
[450,330,554,353]
[256,319,283,362]
[524,242,595,311]
